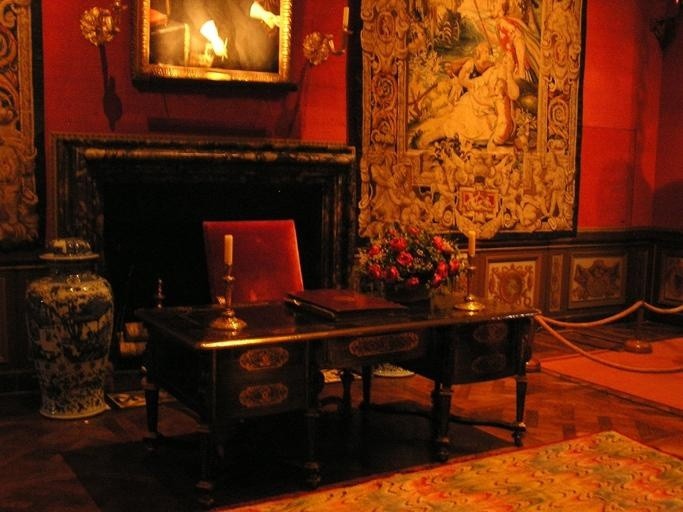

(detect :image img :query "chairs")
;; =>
[205,219,374,418]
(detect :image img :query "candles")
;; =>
[225,234,232,266]
[468,228,475,258]
[343,6,349,28]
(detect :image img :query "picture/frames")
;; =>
[130,0,292,98]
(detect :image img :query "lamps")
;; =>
[81,1,128,131]
[288,30,331,138]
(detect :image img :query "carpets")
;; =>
[211,429,683,512]
[536,333,683,417]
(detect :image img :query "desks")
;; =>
[133,287,542,508]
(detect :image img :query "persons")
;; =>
[544,153,567,218]
[432,142,458,192]
[409,42,518,149]
[494,0,540,78]
[388,164,423,226]
[370,150,398,219]
[472,79,514,149]
[183,1,279,72]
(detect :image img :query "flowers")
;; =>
[353,221,475,299]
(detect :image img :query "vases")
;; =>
[404,299,432,318]
